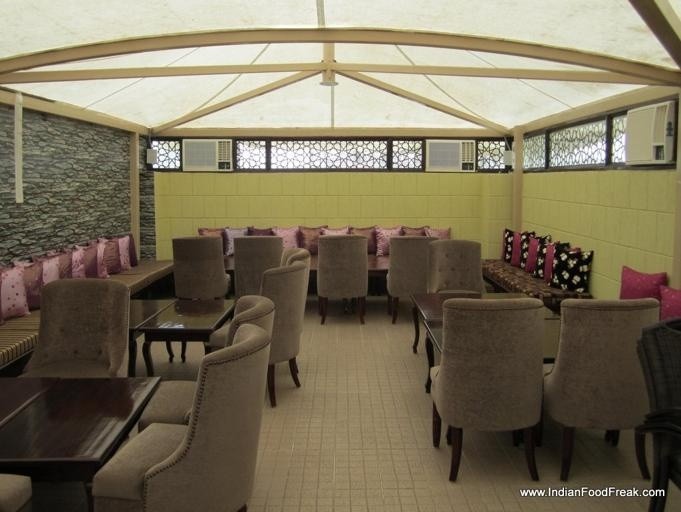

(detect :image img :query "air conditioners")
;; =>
[421,139,476,172]
[181,138,233,173]
[623,99,674,164]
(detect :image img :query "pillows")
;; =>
[197,225,451,255]
